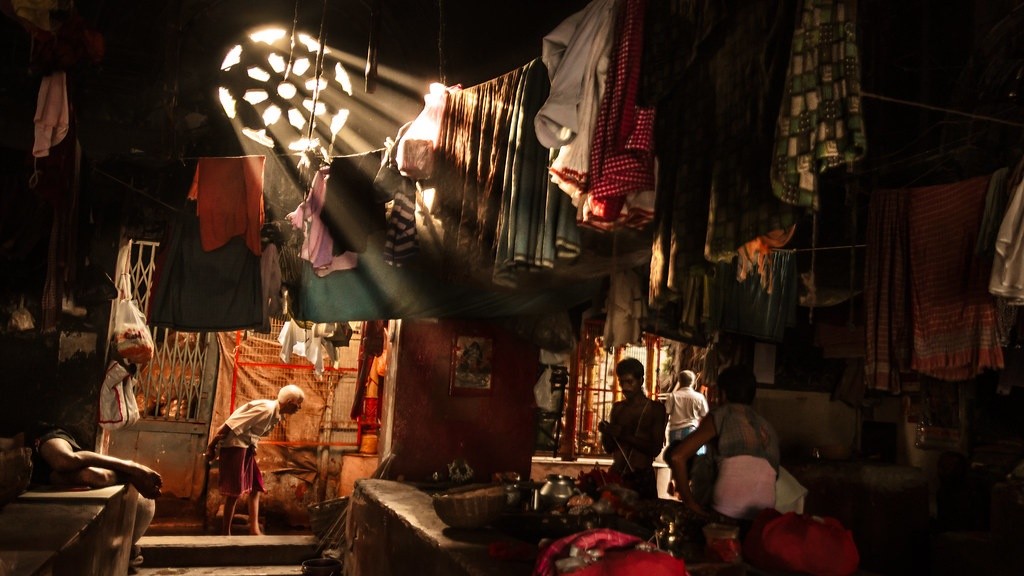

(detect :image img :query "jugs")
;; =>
[537,473,584,512]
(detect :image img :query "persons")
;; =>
[206,383,306,535]
[596,357,667,498]
[660,369,710,497]
[672,365,787,575]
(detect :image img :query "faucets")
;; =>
[652,503,685,550]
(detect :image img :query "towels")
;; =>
[30,71,70,159]
[986,179,1024,306]
